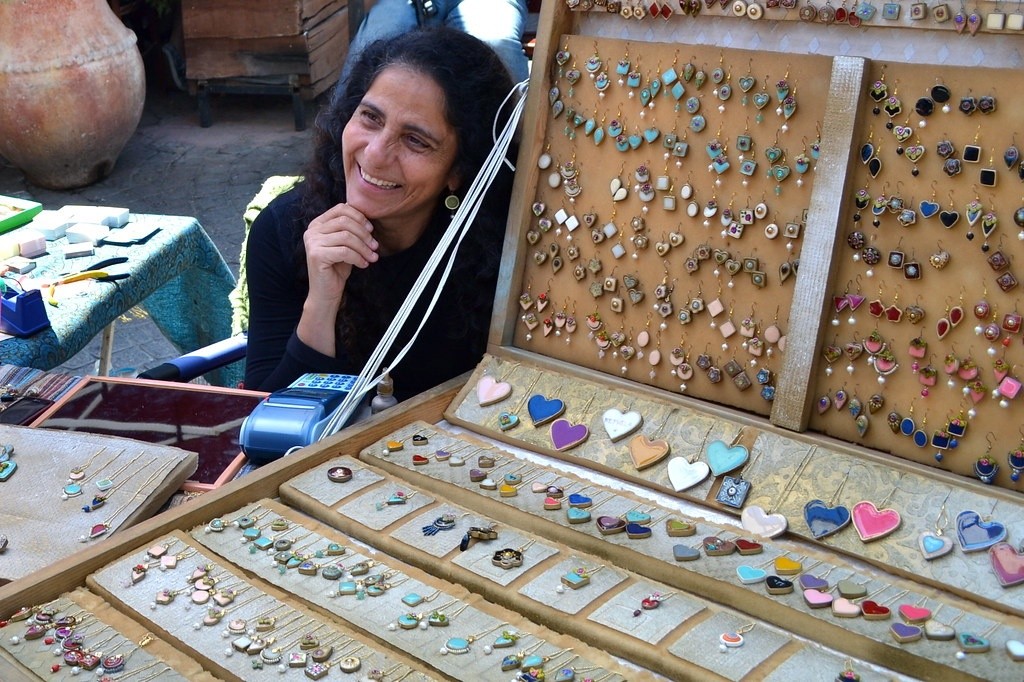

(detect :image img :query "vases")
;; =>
[0,0,146,190]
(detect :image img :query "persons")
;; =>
[242,27,520,402]
[327,0,529,110]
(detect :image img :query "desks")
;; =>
[0,210,249,388]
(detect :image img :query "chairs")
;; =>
[139,176,307,383]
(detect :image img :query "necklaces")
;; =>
[1,364,1024,682]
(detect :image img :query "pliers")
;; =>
[62,256,131,282]
[40,271,110,307]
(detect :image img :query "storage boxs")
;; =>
[0,0,1024,682]
